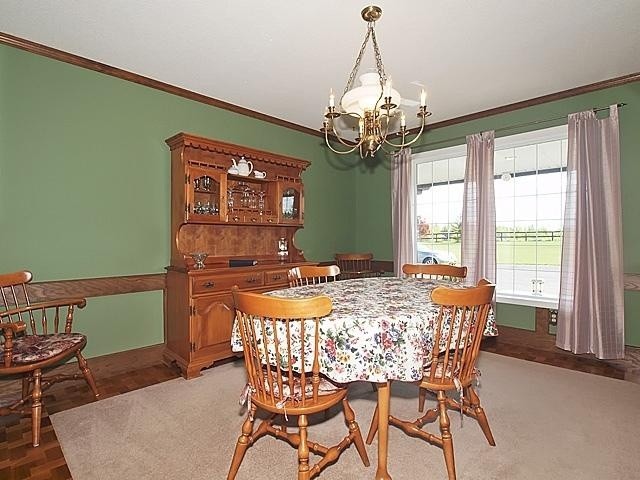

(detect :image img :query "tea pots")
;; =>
[253,170,267,179]
[227,156,254,178]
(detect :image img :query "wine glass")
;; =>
[228,182,267,215]
[193,174,219,216]
[189,252,210,271]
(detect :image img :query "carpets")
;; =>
[47,348,640,480]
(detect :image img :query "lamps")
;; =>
[320,6,433,163]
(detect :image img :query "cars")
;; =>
[417,245,459,266]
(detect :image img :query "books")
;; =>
[229,259,258,266]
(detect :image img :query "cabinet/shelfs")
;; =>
[160,130,312,379]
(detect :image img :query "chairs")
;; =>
[400,262,470,414]
[285,263,342,420]
[228,281,373,480]
[229,276,500,480]
[338,268,386,393]
[366,277,497,480]
[335,250,374,270]
[1,269,101,448]
